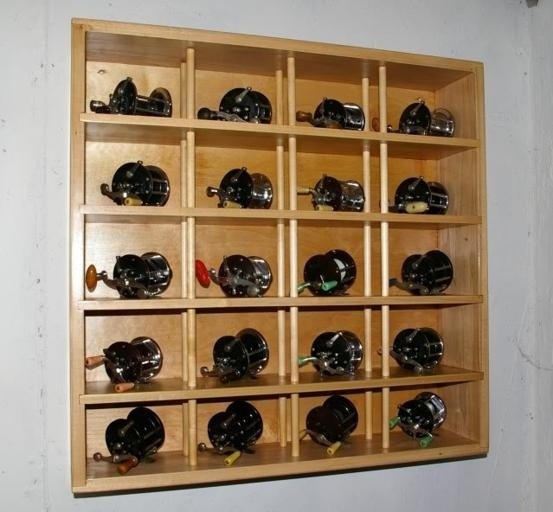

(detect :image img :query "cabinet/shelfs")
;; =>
[68,14,491,497]
